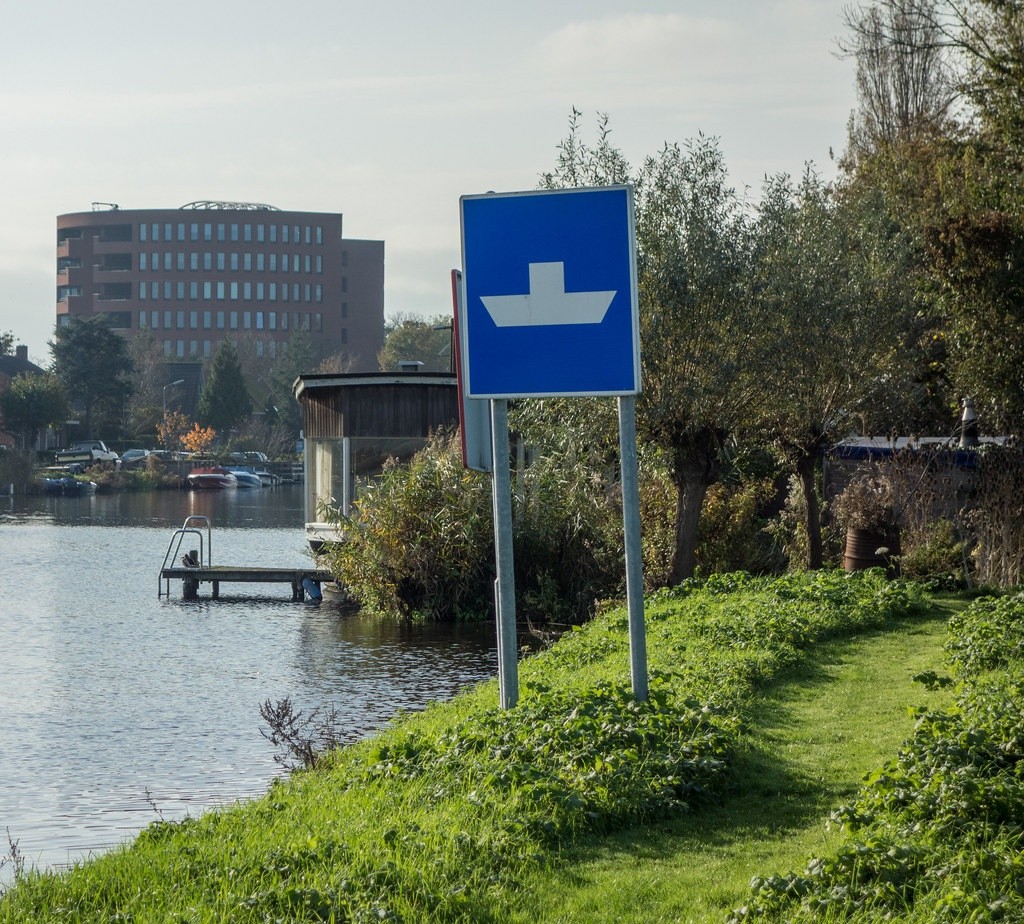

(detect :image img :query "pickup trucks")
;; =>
[55,441,122,473]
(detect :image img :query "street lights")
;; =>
[161,377,186,450]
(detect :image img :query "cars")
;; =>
[178,451,195,460]
[243,451,268,463]
[149,450,183,470]
[229,451,247,464]
[190,451,219,462]
[120,448,150,470]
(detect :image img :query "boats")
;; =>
[292,362,463,552]
[253,467,284,487]
[292,462,305,484]
[229,466,263,489]
[186,466,239,489]
[37,477,98,496]
